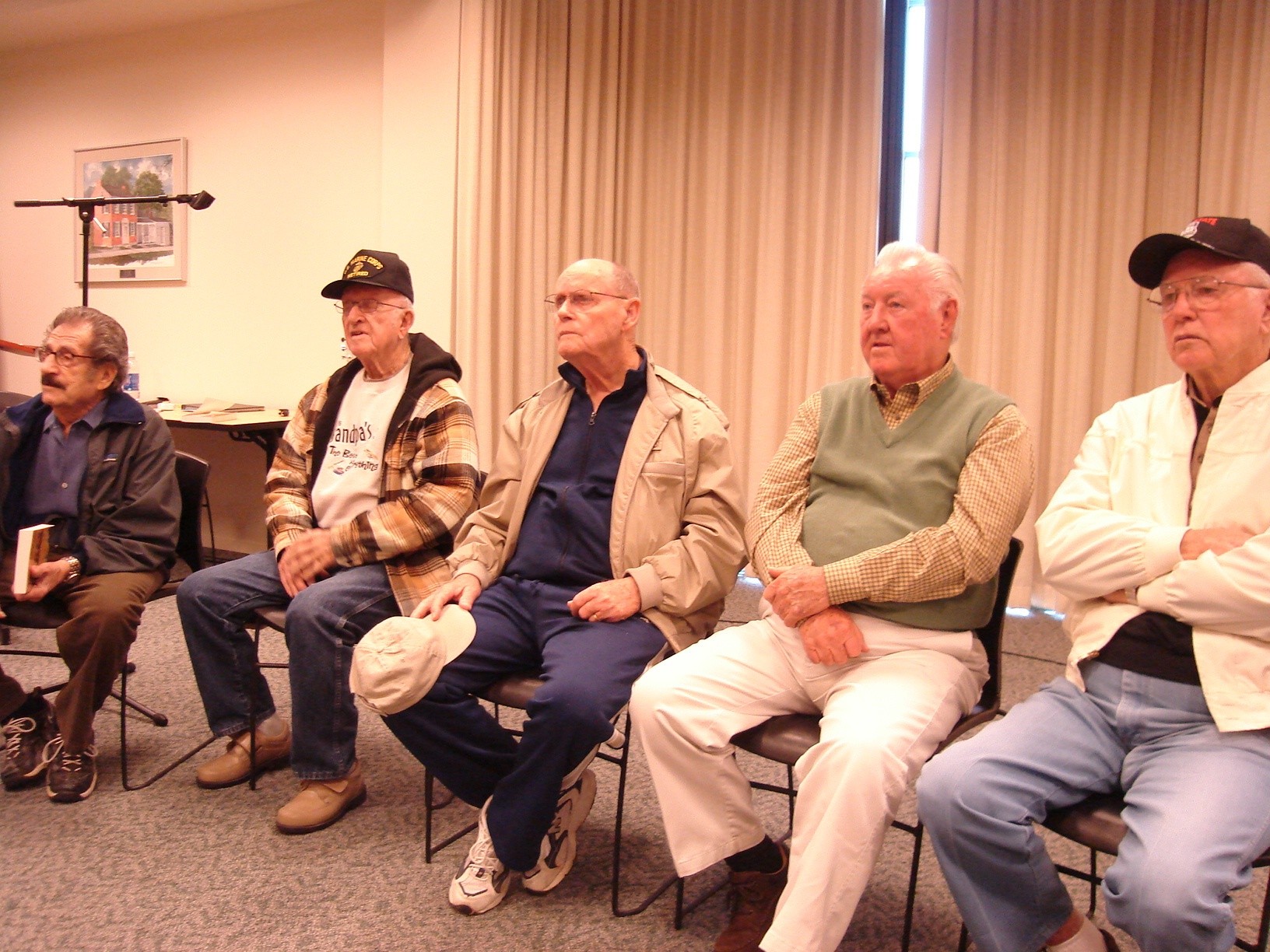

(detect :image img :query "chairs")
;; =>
[956,790,1270,952]
[672,536,1023,952]
[424,629,715,917]
[0,449,217,790]
[249,471,488,810]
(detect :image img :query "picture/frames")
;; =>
[74,136,188,280]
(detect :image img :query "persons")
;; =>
[379,259,751,916]
[174,249,481,833]
[917,217,1270,952]
[0,306,181,801]
[629,240,1036,952]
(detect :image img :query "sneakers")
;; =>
[24,734,101,801]
[521,767,597,895]
[1,691,56,783]
[445,793,514,914]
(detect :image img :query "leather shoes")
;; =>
[276,767,367,835]
[714,845,790,952]
[193,721,292,789]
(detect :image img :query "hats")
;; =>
[1130,212,1269,288]
[346,602,476,718]
[321,250,415,303]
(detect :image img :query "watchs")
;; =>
[61,556,81,584]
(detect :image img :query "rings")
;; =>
[594,614,598,621]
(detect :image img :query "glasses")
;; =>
[1144,275,1266,307]
[544,289,627,308]
[31,346,96,369]
[331,297,404,314]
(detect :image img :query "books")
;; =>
[14,524,55,596]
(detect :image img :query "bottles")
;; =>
[121,351,140,402]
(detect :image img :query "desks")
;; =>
[137,399,295,566]
